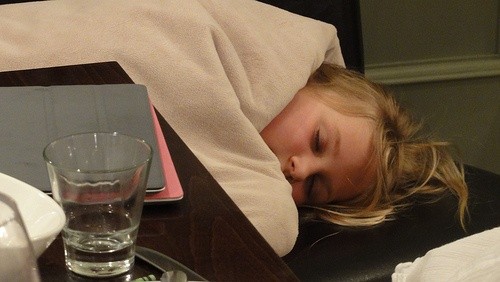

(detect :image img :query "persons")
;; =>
[258,61,469,228]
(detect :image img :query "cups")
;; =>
[41,132,154,278]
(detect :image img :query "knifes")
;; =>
[135,245,209,282]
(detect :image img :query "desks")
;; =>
[0,60,303,282]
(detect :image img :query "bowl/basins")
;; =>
[0,174,67,282]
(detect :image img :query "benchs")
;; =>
[257,0,500,282]
